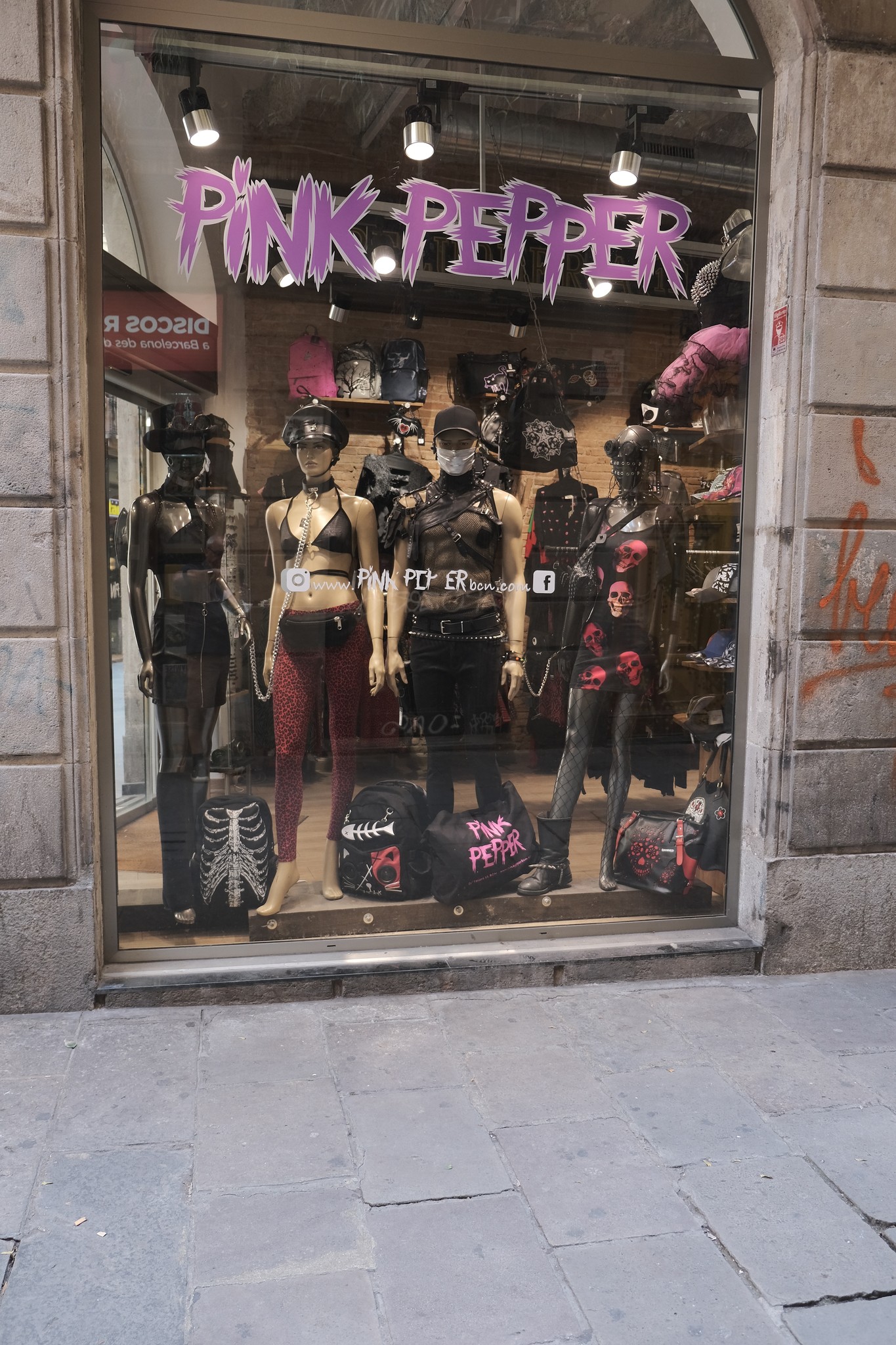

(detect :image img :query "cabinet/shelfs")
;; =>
[195,474,257,816]
[612,362,750,820]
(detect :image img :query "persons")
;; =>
[517,424,685,895]
[384,405,527,814]
[256,404,385,916]
[129,401,253,922]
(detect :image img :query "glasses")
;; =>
[164,414,211,431]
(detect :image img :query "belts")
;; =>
[411,613,500,635]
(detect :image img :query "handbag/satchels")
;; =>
[682,742,728,877]
[457,348,526,396]
[551,358,609,396]
[521,356,565,397]
[686,693,732,734]
[277,610,355,650]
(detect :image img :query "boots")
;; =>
[518,810,573,894]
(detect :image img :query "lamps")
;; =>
[502,318,533,342]
[267,257,297,297]
[394,102,442,166]
[365,235,400,284]
[321,299,349,328]
[600,131,657,188]
[580,262,615,307]
[166,89,225,149]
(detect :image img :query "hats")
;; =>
[685,563,738,602]
[142,402,217,452]
[282,403,349,452]
[690,464,743,502]
[432,405,479,439]
[687,627,736,669]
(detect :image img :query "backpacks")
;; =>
[421,780,540,905]
[288,324,339,398]
[192,796,274,913]
[340,780,431,901]
[334,339,381,400]
[379,337,430,403]
[620,809,705,898]
